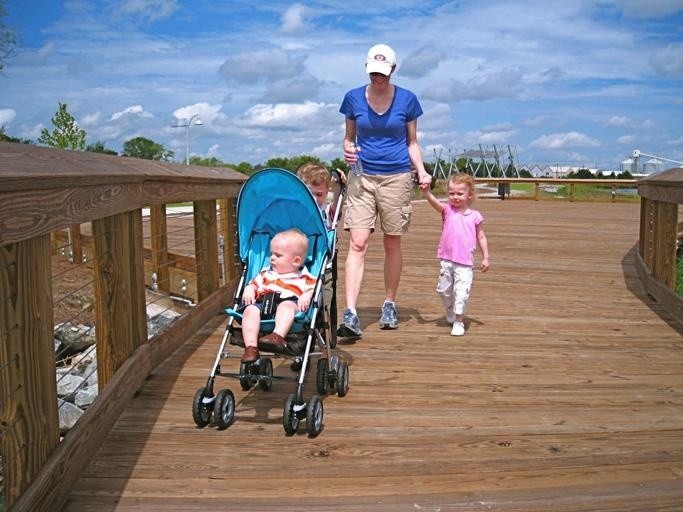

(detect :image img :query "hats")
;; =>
[366,44,396,77]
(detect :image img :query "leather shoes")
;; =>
[242,333,286,363]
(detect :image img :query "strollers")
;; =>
[189,164,353,438]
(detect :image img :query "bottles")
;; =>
[348,142,363,176]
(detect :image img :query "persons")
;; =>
[335,41,434,339]
[417,173,491,338]
[233,228,320,365]
[291,162,349,346]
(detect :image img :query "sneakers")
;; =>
[379,302,399,330]
[291,357,310,371]
[446,310,465,336]
[337,309,362,337]
